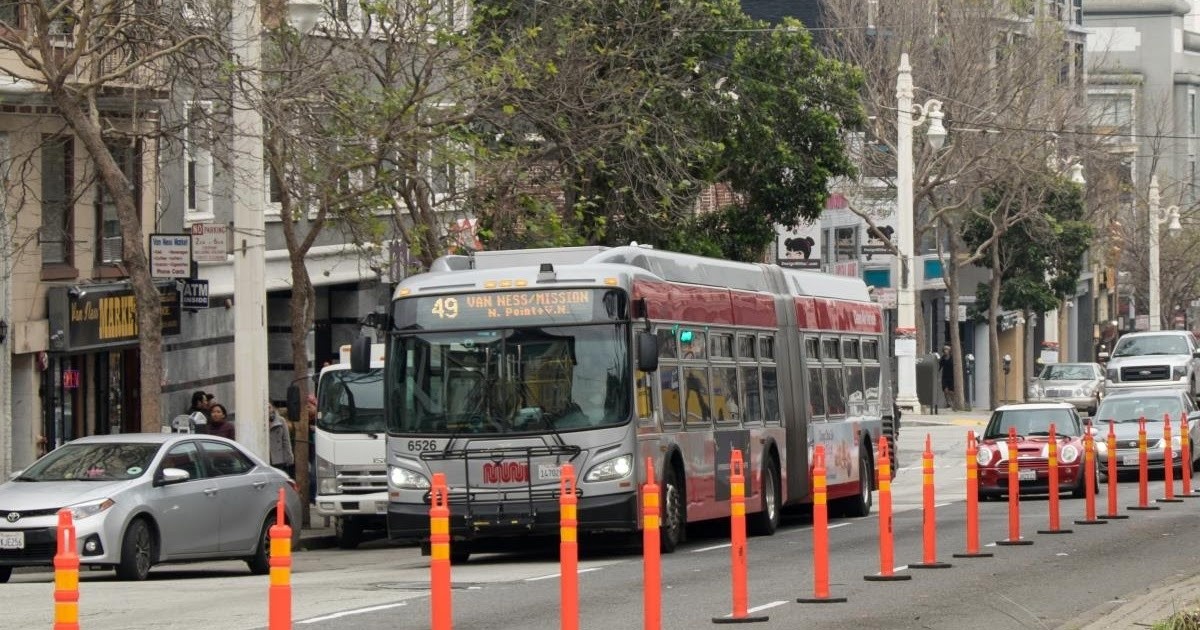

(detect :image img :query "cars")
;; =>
[1028,363,1107,417]
[974,401,1098,502]
[0,435,302,582]
[1082,389,1200,479]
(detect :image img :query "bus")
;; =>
[350,241,890,556]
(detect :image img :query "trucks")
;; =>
[286,341,482,550]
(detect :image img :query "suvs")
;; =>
[1098,330,1200,397]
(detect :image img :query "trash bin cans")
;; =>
[916,354,939,405]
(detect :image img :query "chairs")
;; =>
[1114,403,1136,419]
[1157,399,1178,419]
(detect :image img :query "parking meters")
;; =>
[931,352,940,413]
[1002,354,1011,404]
[964,354,974,410]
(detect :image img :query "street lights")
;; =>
[1148,176,1183,334]
[234,1,325,465]
[895,53,948,415]
[1042,131,1087,379]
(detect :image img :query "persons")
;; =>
[188,391,217,434]
[938,346,954,408]
[205,404,234,440]
[269,397,294,470]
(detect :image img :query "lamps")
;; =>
[69,285,87,300]
